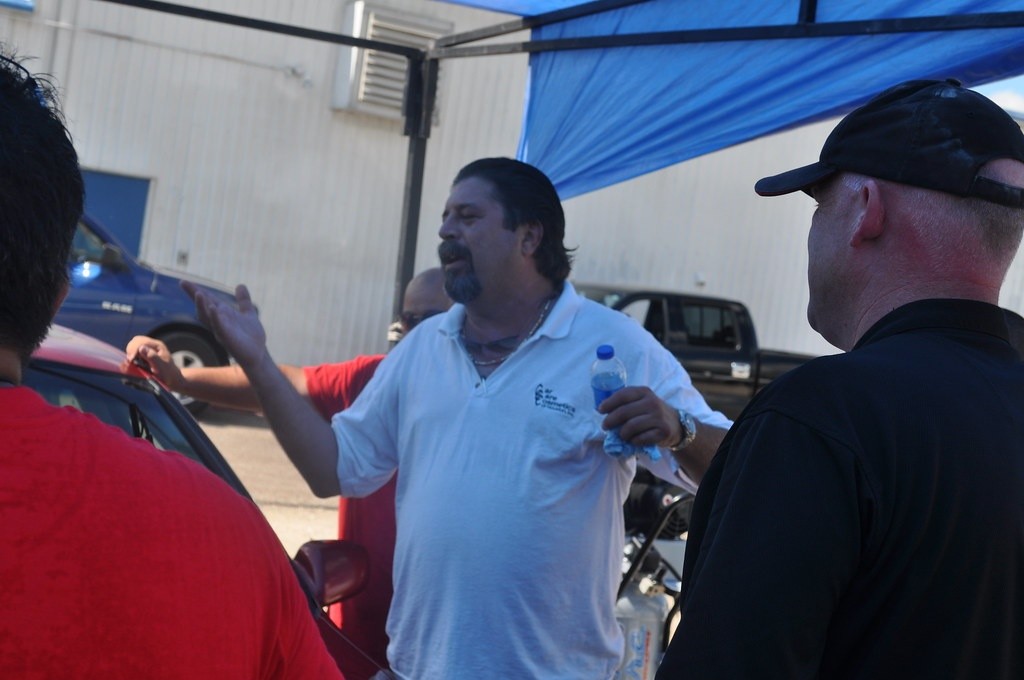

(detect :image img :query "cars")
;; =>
[26,321,390,678]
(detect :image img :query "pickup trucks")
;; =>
[604,287,823,425]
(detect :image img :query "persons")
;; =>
[655,79,1023,679]
[181,157,732,680]
[126,268,457,680]
[0,52,344,679]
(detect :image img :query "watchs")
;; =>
[668,409,696,451]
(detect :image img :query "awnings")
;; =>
[101,0,1023,355]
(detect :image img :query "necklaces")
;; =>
[461,299,552,366]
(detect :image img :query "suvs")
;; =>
[51,209,252,421]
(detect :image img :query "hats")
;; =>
[755,78,1024,210]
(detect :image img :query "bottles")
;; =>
[591,344,628,434]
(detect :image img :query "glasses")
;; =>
[401,310,446,330]
